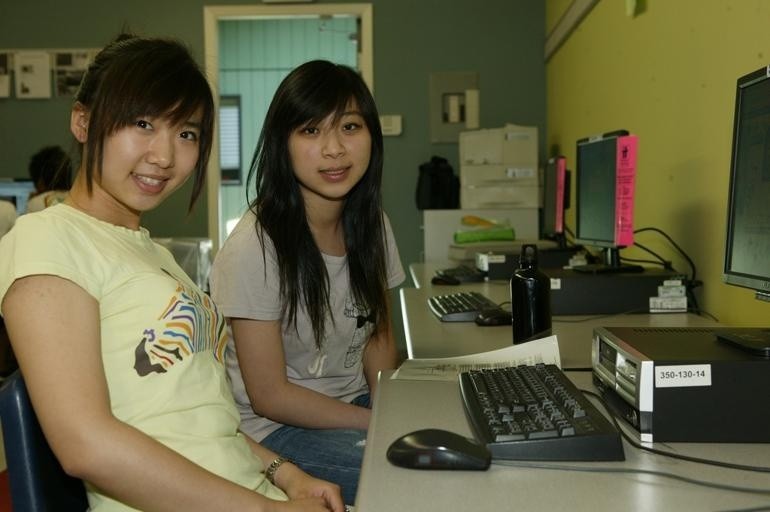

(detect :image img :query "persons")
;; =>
[207,61,405,507]
[26,145,73,215]
[2,36,345,512]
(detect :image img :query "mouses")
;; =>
[432,276,461,286]
[476,310,512,327]
[386,428,492,472]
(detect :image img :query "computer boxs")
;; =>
[591,325,768,443]
[535,268,687,317]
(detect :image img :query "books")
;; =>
[0,49,92,99]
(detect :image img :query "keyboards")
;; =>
[459,364,625,462]
[427,291,504,322]
[435,265,484,283]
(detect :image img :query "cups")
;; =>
[510,244,552,344]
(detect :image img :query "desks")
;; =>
[356,370,770,512]
[409,263,453,288]
[400,279,729,369]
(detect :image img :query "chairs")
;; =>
[0,367,89,511]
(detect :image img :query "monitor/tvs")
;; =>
[542,156,571,248]
[723,65,770,303]
[573,129,644,272]
